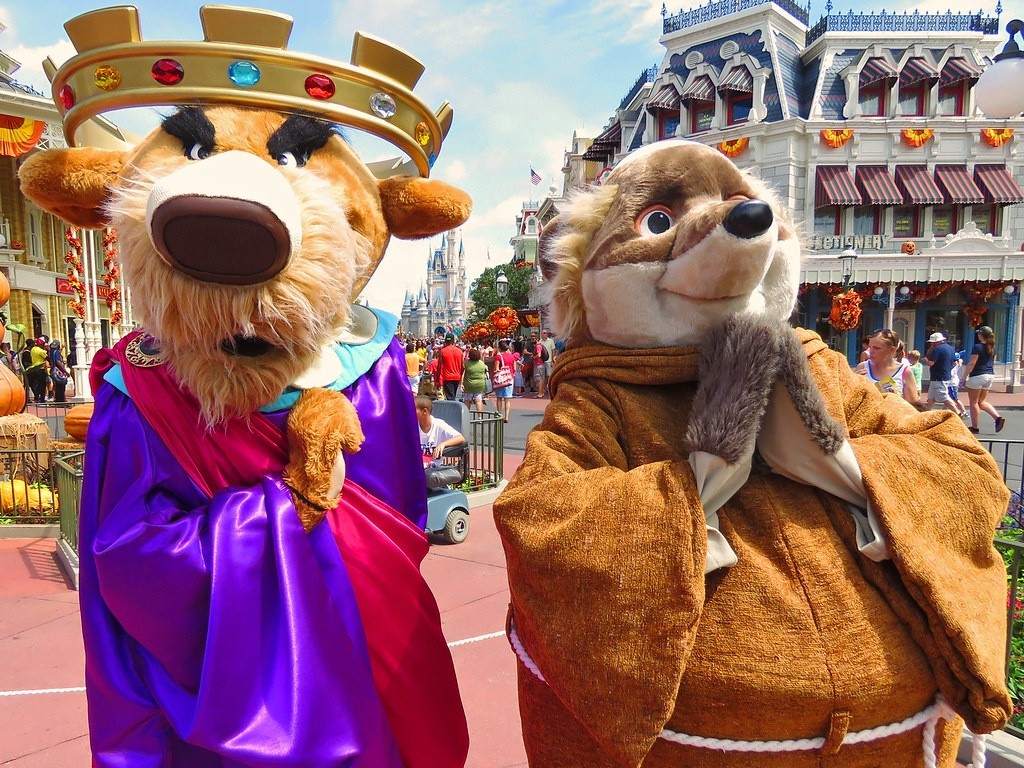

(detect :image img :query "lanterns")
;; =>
[902,240,915,255]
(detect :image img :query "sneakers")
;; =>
[994,417,1005,433]
[967,427,979,434]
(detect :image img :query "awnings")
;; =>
[646,66,753,117]
[895,165,944,209]
[856,166,904,209]
[582,121,621,163]
[858,57,980,90]
[935,164,985,209]
[974,164,1024,208]
[815,165,862,211]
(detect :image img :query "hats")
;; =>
[51,340,60,349]
[34,337,45,346]
[976,326,992,334]
[445,334,456,341]
[926,332,947,342]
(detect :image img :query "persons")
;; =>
[495,340,516,423]
[398,330,557,400]
[854,329,970,420]
[1,335,77,409]
[958,326,1006,434]
[437,334,465,401]
[460,349,488,424]
[414,395,465,469]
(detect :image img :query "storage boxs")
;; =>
[0,413,49,484]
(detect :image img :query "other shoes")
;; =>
[530,389,539,393]
[958,412,970,420]
[478,419,482,424]
[517,390,526,394]
[512,393,523,398]
[504,420,509,423]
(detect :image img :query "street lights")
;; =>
[838,243,858,360]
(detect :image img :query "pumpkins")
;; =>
[0,474,59,518]
[64,403,94,442]
[0,272,25,418]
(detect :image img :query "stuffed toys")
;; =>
[17,5,470,768]
[490,139,1015,768]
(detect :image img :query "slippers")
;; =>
[531,394,544,399]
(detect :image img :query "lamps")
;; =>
[895,287,910,304]
[871,286,889,305]
[1001,286,1016,302]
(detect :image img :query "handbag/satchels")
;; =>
[50,367,68,385]
[491,366,512,391]
[485,378,494,394]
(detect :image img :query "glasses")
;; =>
[873,328,895,346]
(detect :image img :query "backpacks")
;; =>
[536,343,549,361]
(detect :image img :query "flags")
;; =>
[530,168,541,185]
